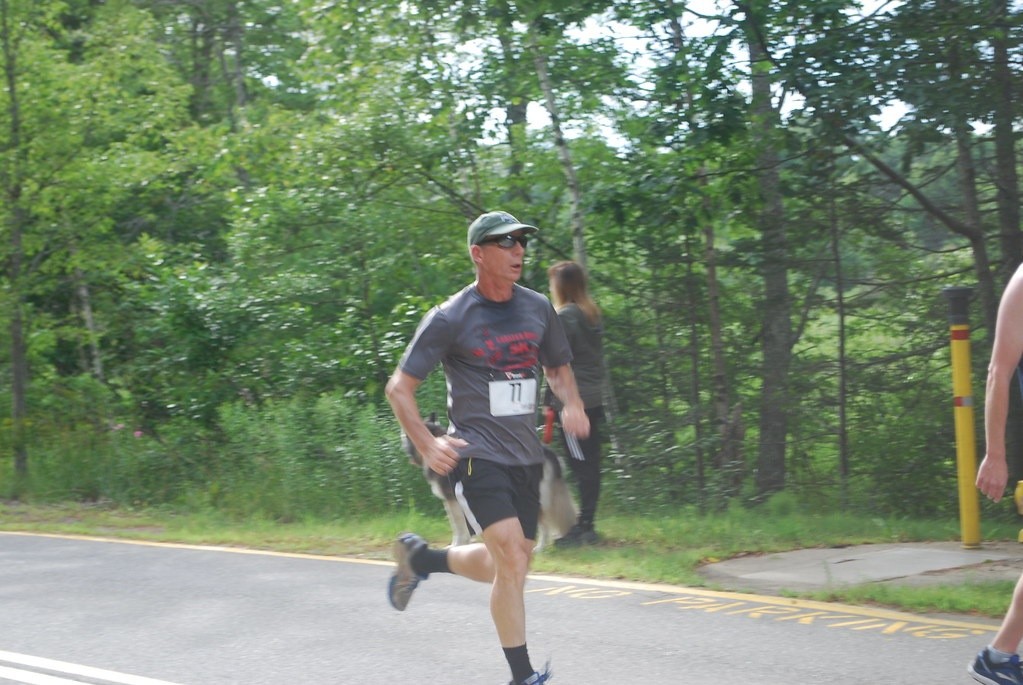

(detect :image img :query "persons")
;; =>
[540,258,606,546]
[388,208,590,685]
[970,259,1023,685]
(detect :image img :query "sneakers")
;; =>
[553,524,597,547]
[386,532,429,614]
[508,672,551,685]
[966,646,1023,685]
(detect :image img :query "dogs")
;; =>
[401,412,578,552]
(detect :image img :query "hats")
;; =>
[466,211,538,249]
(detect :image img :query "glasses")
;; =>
[478,235,528,249]
